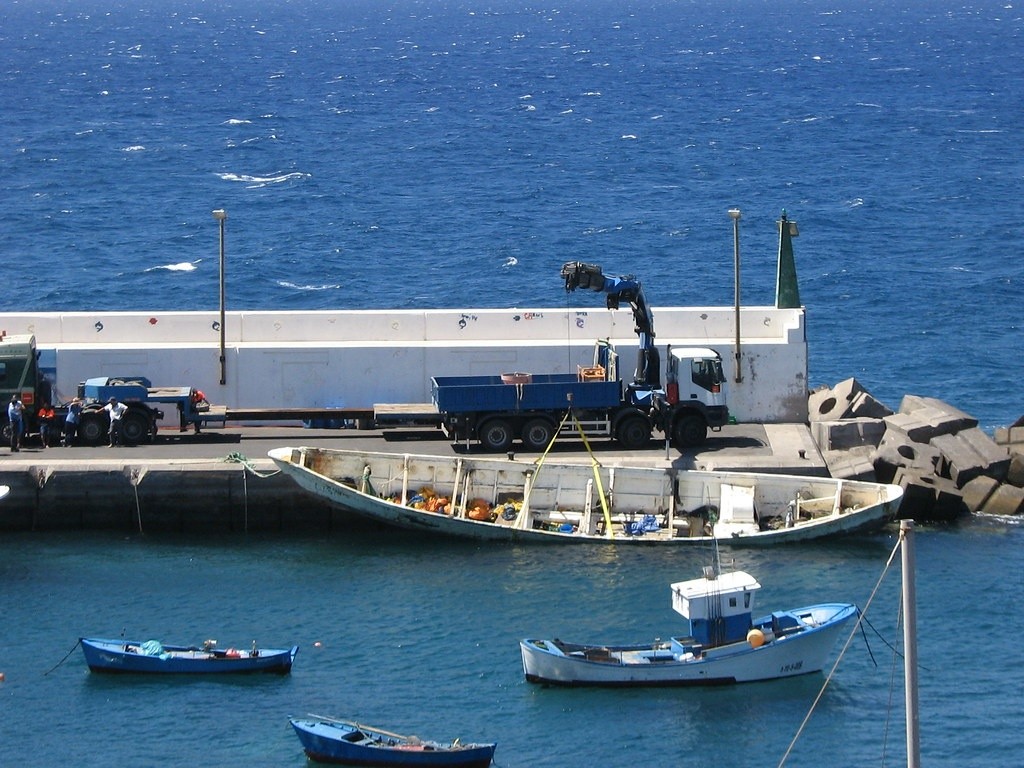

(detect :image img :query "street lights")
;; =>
[212,209,227,385]
[727,207,743,384]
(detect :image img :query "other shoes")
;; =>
[40,445,45,449]
[46,445,49,449]
[108,443,115,448]
[117,442,120,447]
[10,447,19,452]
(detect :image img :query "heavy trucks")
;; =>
[0,330,228,447]
[429,261,730,453]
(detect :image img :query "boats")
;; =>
[519,566,859,688]
[266,445,906,549]
[287,712,499,768]
[76,636,300,674]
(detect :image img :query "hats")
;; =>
[108,397,116,403]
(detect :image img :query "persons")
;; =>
[190,388,210,435]
[7,394,129,453]
